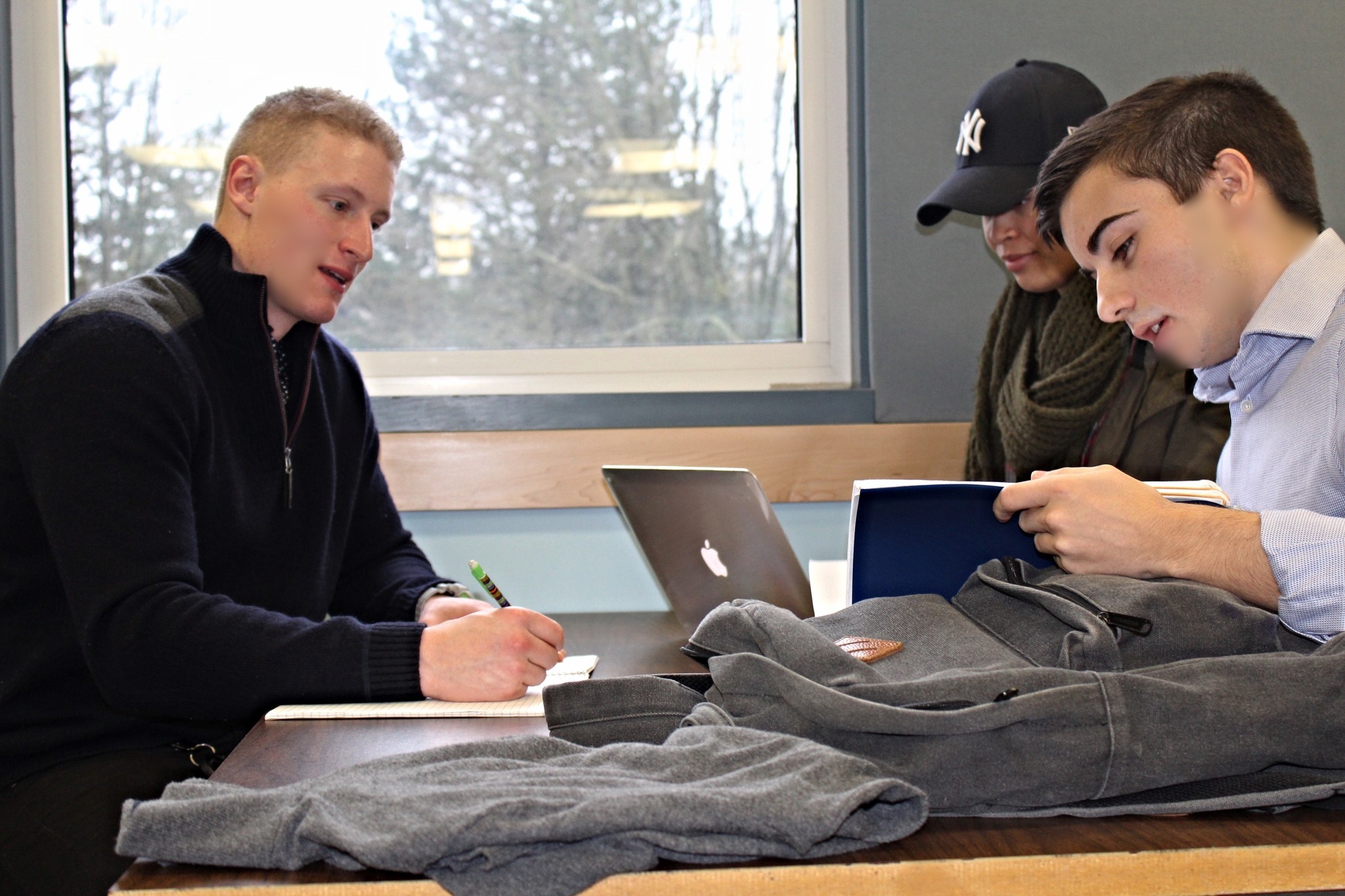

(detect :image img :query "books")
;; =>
[264,653,603,723]
[850,478,1228,620]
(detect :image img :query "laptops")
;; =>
[602,464,814,634]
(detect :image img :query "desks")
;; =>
[108,612,1345,896]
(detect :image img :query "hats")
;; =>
[916,59,1108,227]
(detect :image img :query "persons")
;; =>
[0,84,565,896]
[988,73,1344,647]
[914,56,1229,490]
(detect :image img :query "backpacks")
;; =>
[545,555,1345,818]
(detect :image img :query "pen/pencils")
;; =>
[466,561,512,611]
